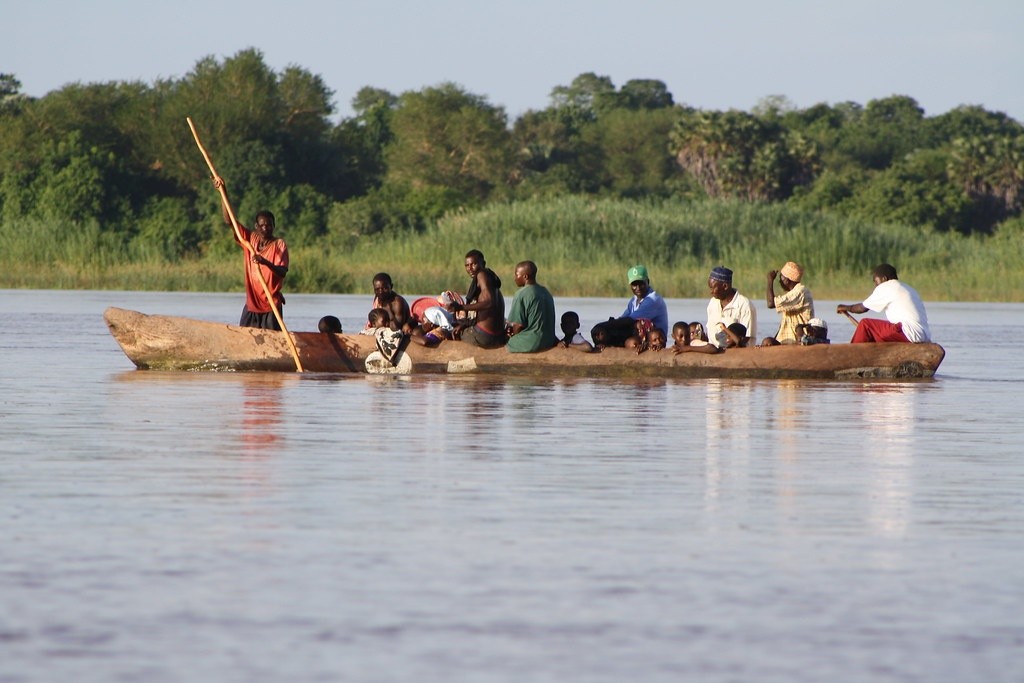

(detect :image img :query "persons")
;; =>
[619,265,668,349]
[209,176,289,332]
[706,267,757,349]
[319,316,342,334]
[591,317,829,356]
[837,263,930,344]
[505,261,555,353]
[447,249,505,348]
[556,311,593,352]
[766,262,814,345]
[368,273,452,346]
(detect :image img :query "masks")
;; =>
[779,278,791,291]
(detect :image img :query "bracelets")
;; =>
[850,305,855,313]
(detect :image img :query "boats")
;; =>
[103,304,944,379]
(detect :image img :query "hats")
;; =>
[709,265,734,283]
[873,263,899,280]
[781,261,804,283]
[627,264,649,284]
[806,317,829,329]
